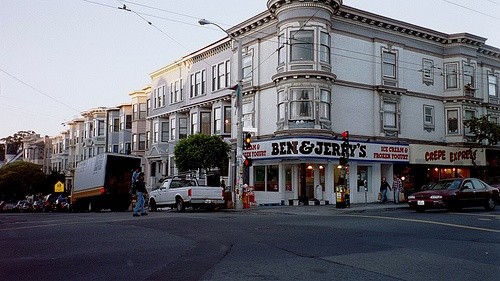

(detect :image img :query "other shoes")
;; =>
[133,213,140,217]
[141,213,148,216]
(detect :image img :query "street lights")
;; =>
[196,18,245,213]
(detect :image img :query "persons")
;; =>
[392,174,402,205]
[221,180,226,189]
[380,177,392,204]
[129,167,148,217]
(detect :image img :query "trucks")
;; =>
[71,152,144,212]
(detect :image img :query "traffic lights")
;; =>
[342,130,349,152]
[243,157,252,166]
[245,133,252,150]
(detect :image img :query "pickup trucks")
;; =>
[148,175,227,212]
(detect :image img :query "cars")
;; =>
[407,177,500,212]
[0,193,71,212]
